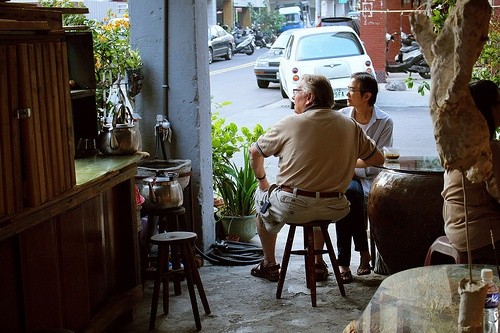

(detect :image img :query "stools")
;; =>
[148,232,211,329]
[277,220,344,306]
[143,207,186,295]
[425,236,498,265]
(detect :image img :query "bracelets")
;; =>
[255,173,266,180]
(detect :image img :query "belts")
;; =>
[281,187,343,198]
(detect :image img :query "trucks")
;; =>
[275,1,310,38]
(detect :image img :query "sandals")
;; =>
[251,259,280,281]
[313,261,328,281]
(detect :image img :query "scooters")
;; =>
[386,32,431,79]
[217,22,266,56]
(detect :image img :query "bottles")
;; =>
[479,268,499,333]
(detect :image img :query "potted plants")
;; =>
[211,95,270,241]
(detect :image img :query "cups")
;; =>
[384,147,401,162]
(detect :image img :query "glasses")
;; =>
[292,89,303,94]
[347,86,363,92]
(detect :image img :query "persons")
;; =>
[249,74,385,282]
[337,72,393,284]
[440,80,500,265]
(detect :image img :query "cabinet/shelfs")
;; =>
[0,32,142,333]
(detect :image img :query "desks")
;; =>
[353,265,500,333]
[368,155,455,274]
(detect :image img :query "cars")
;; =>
[253,12,360,88]
[208,24,235,64]
[273,30,376,110]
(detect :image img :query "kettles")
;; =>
[98,103,139,156]
[141,168,184,208]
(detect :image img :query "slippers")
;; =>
[357,254,371,274]
[341,270,352,283]
